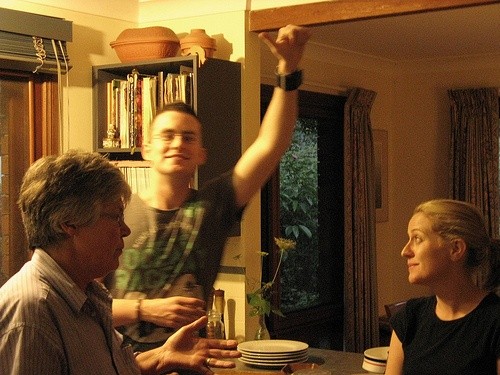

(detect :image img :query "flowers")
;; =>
[233,236,296,326]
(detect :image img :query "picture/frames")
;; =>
[371,128,389,223]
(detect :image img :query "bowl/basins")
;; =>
[362,347,391,374]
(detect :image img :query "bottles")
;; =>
[206,309,225,341]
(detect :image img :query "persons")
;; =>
[94,23,312,375]
[0,149,241,375]
[385,198,500,375]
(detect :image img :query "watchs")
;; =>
[274,68,304,91]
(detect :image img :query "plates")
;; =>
[236,340,309,369]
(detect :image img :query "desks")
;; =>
[185,347,383,375]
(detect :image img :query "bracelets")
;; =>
[135,298,142,323]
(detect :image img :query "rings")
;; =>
[206,358,211,366]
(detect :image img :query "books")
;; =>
[103,65,194,203]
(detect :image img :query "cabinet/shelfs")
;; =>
[92,54,241,240]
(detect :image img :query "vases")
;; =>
[256,310,271,340]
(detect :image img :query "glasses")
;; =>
[148,130,203,144]
[95,207,125,227]
